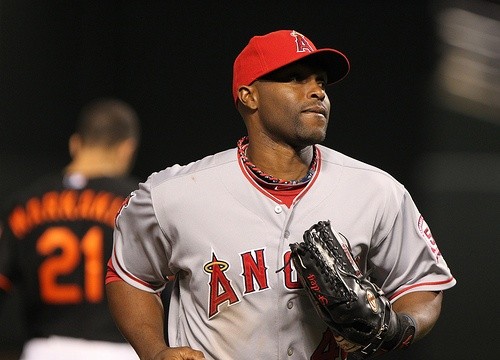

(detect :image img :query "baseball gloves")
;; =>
[289,218,393,354]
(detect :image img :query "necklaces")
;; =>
[237,135,318,184]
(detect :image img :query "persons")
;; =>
[0,98,175,360]
[105,29,457,360]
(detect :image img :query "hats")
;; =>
[232,31,350,110]
